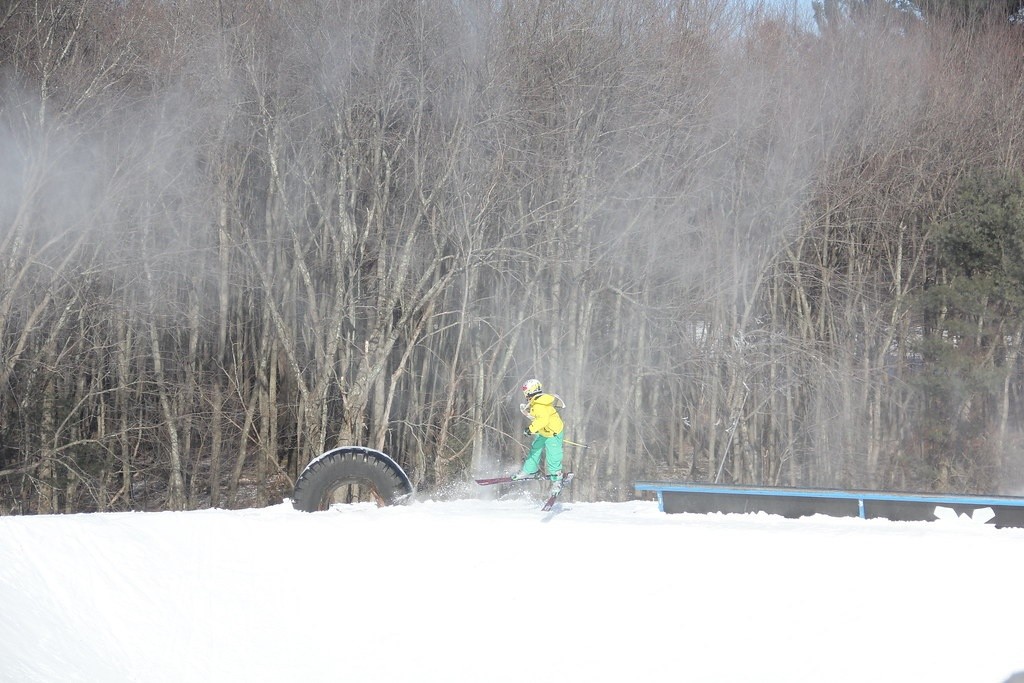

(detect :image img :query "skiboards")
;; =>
[474,471,575,512]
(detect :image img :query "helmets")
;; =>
[522,379,542,398]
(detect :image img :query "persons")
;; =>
[512,379,565,494]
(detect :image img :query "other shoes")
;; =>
[511,471,536,480]
[551,479,563,493]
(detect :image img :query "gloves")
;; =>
[524,428,532,437]
